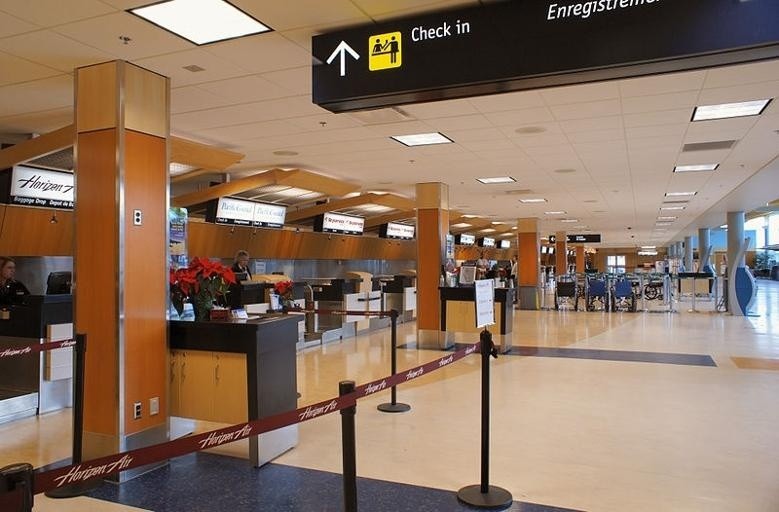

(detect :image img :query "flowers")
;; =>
[167,254,236,318]
[274,280,295,296]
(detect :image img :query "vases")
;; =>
[191,288,214,323]
[278,292,290,307]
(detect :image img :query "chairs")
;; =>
[554,270,663,313]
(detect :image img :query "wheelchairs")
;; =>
[553,272,664,312]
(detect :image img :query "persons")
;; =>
[511,254,518,305]
[477,249,489,281]
[0,256,30,305]
[230,250,252,282]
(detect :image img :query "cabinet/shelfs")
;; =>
[167,348,214,423]
[211,349,249,426]
